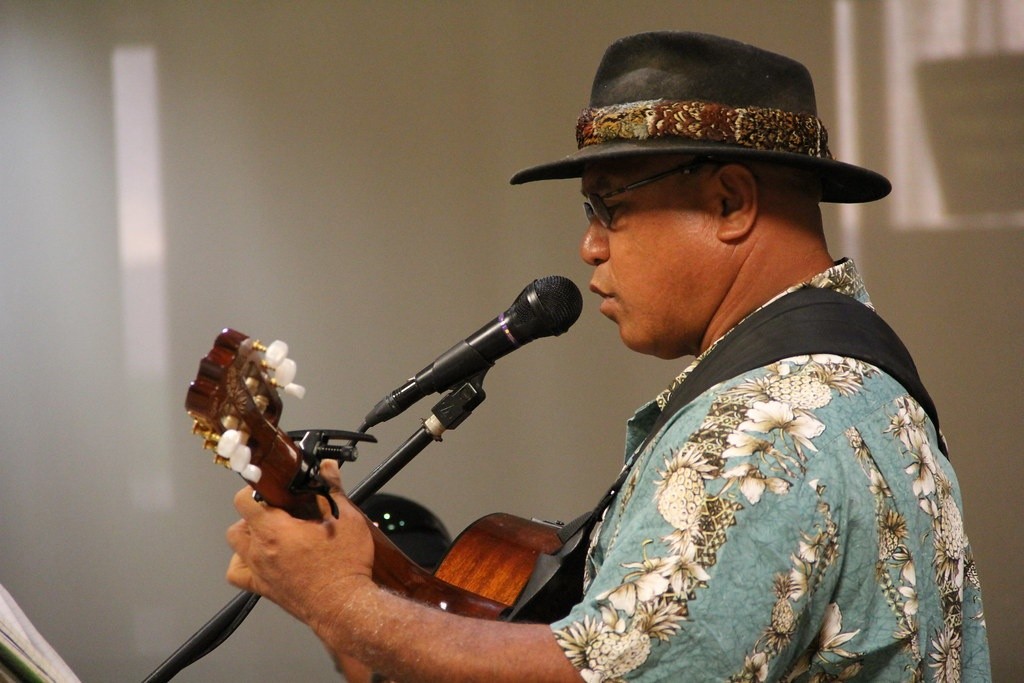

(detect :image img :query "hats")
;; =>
[511,31,892,204]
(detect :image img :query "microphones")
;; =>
[366,274,583,428]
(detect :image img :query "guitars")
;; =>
[184,326,568,683]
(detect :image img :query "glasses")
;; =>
[582,156,709,228]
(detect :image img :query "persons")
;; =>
[325,492,452,683]
[228,32,991,683]
